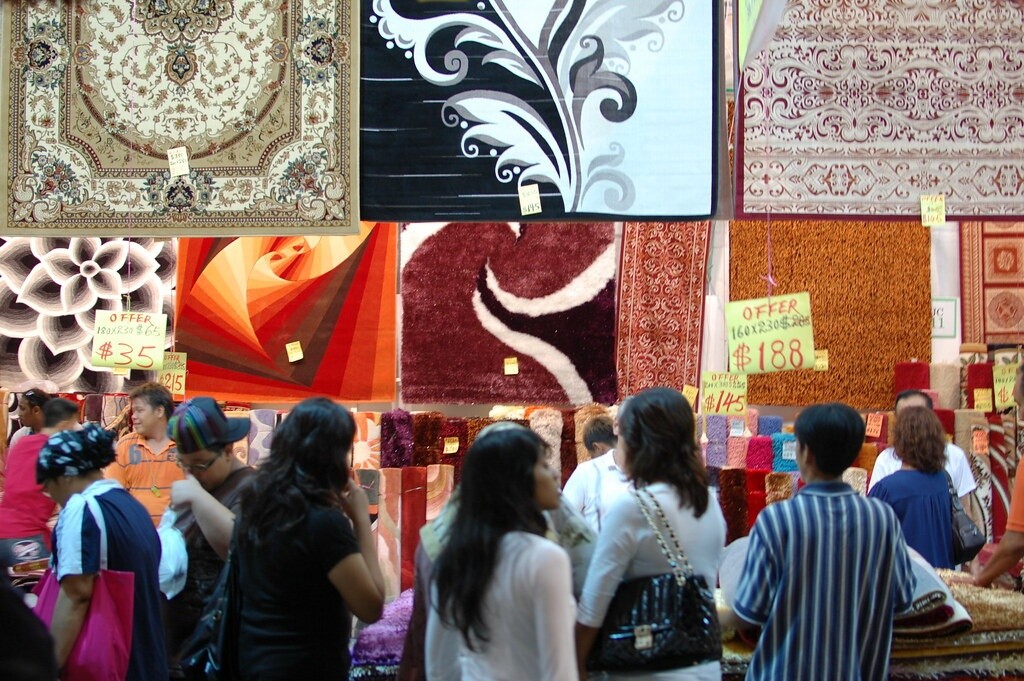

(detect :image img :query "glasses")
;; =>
[175,453,221,473]
[38,474,53,498]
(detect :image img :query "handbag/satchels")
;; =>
[943,469,986,566]
[586,485,724,675]
[30,494,132,681]
[156,507,189,601]
[177,545,244,680]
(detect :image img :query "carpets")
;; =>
[0,0,1024,681]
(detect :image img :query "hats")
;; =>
[166,396,252,455]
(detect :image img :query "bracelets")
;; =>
[4,402,8,405]
[972,577,977,586]
[0,402,3,404]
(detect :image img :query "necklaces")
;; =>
[143,447,165,497]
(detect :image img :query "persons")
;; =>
[866,389,977,570]
[730,402,917,681]
[948,363,1024,587]
[232,396,386,681]
[0,381,256,681]
[396,387,727,681]
